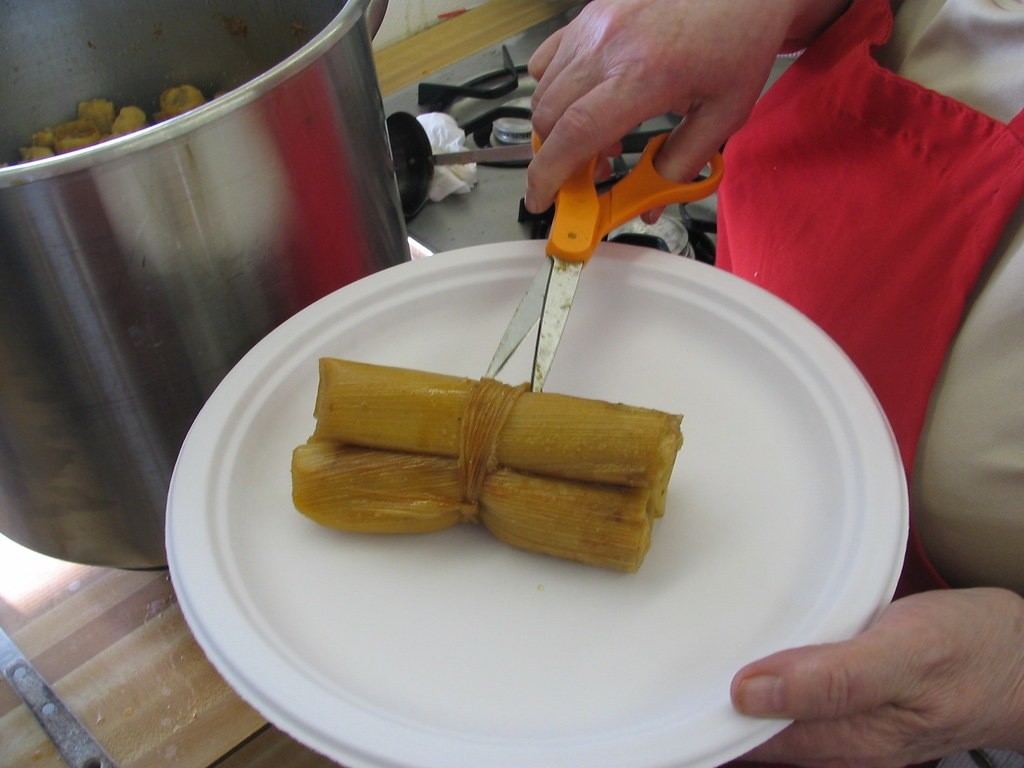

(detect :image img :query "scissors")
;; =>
[476,122,728,395]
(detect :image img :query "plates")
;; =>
[164,239,909,768]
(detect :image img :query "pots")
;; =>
[0,0,412,578]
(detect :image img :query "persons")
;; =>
[522,0,1024,768]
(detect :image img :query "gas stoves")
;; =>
[381,0,810,266]
